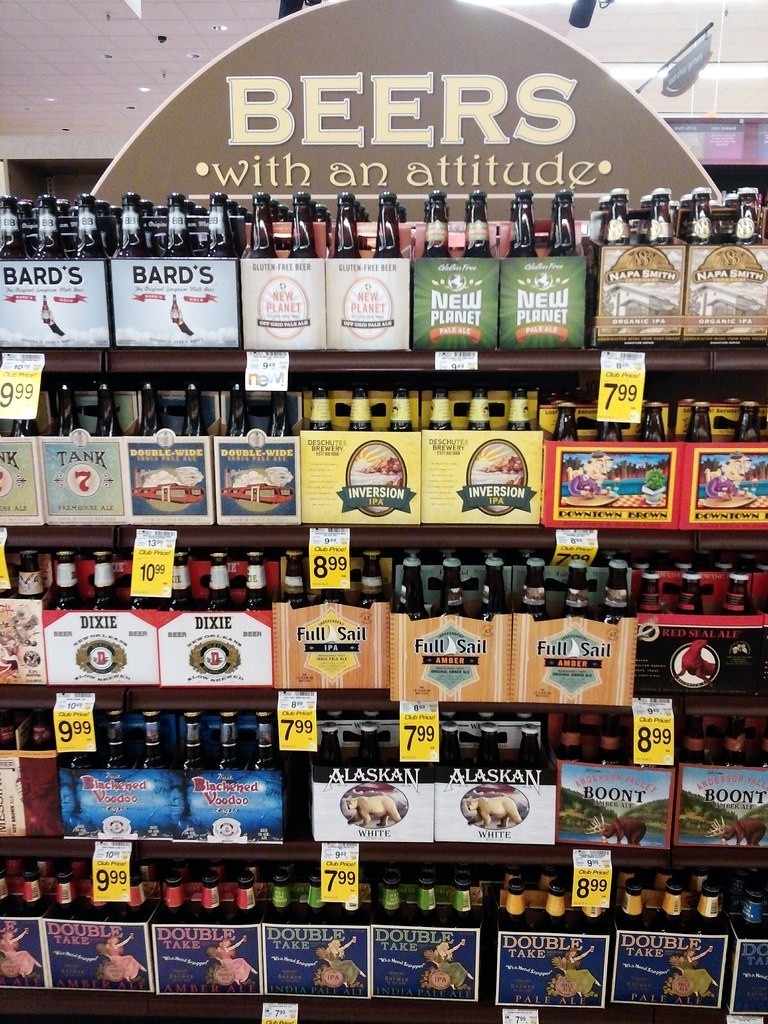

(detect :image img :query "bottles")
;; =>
[0,859,768,936]
[0,707,767,770]
[0,546,768,623]
[0,185,768,263]
[0,378,767,441]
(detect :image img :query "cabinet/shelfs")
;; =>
[1,194,768,1024]
[0,345,768,1024]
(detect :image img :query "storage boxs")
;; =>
[0,916,768,1015]
[0,259,768,348]
[2,748,768,851]
[1,598,768,709]
[0,435,767,532]
